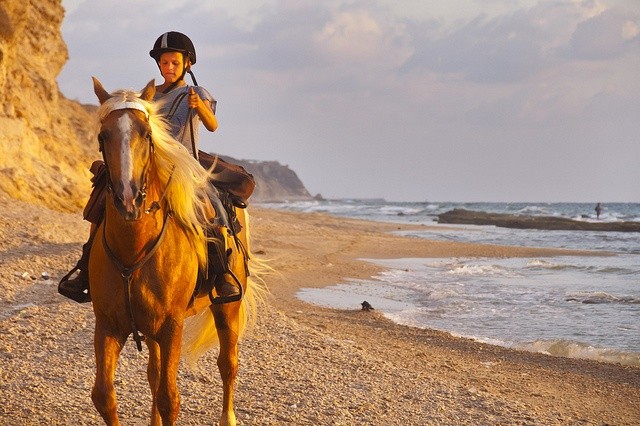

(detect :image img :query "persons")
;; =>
[60,30,239,302]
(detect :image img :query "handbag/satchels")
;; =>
[83,158,110,223]
[199,151,256,209]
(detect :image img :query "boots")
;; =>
[59,241,98,296]
[207,248,241,298]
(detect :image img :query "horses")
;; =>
[87,75,289,425]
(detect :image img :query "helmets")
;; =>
[149,32,196,64]
